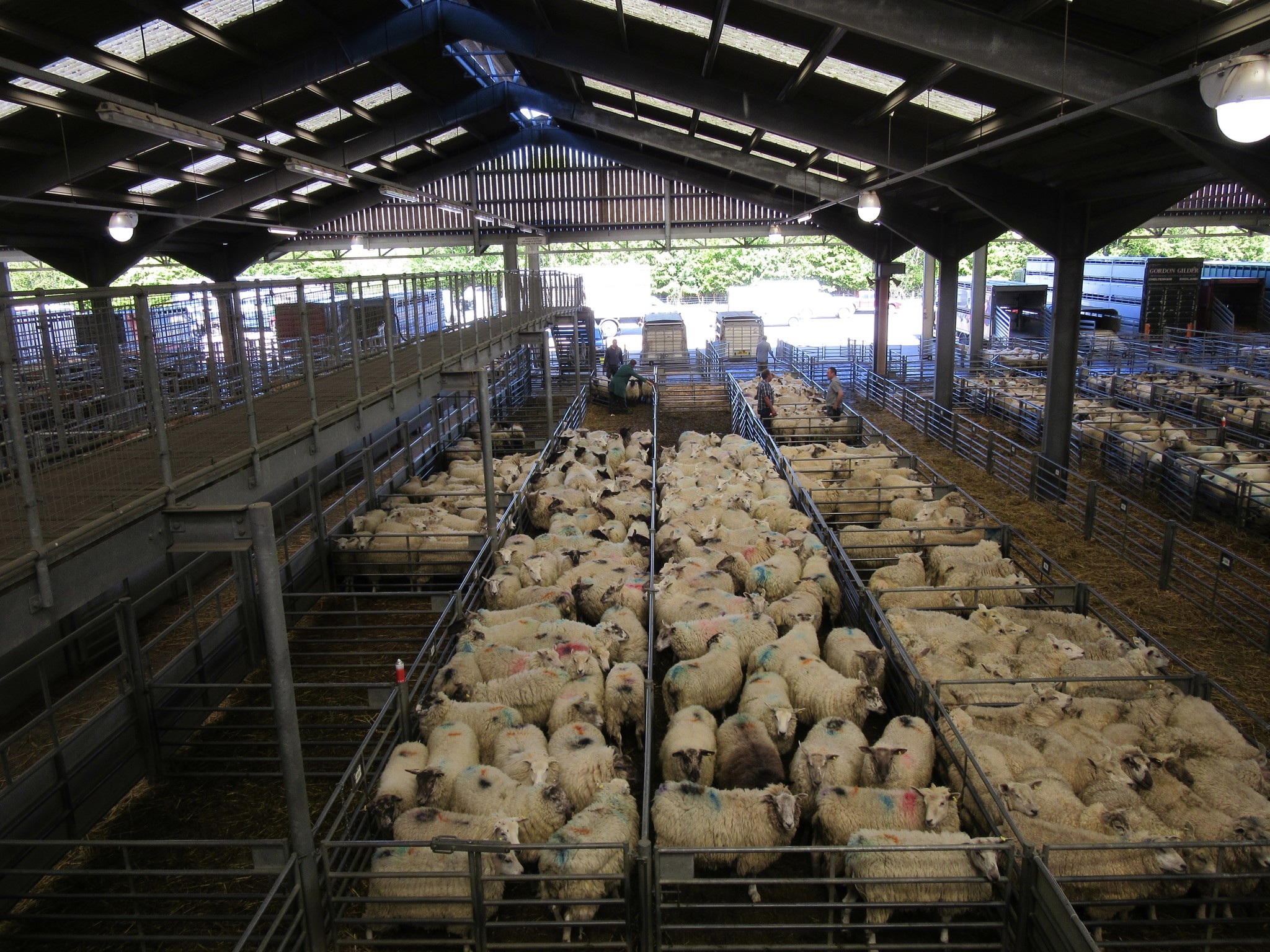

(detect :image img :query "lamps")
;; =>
[857,191,882,223]
[1199,47,1270,143]
[107,212,138,242]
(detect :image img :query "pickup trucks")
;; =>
[830,290,902,316]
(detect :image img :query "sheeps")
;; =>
[405,724,484,812]
[375,742,423,831]
[840,828,1008,945]
[590,375,653,405]
[959,327,1268,519]
[420,428,653,808]
[334,447,541,575]
[452,766,566,866]
[859,537,1046,606]
[657,704,718,790]
[880,601,1177,701]
[716,716,784,791]
[448,418,525,458]
[732,373,847,446]
[535,775,642,947]
[654,426,884,743]
[938,682,1270,936]
[788,715,872,814]
[781,431,979,545]
[365,806,528,947]
[872,712,938,790]
[817,779,963,875]
[492,722,553,791]
[646,781,809,905]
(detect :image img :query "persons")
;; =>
[757,369,777,438]
[756,335,776,377]
[640,350,643,366]
[609,359,654,415]
[825,367,844,422]
[603,339,624,380]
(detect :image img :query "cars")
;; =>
[565,325,608,362]
